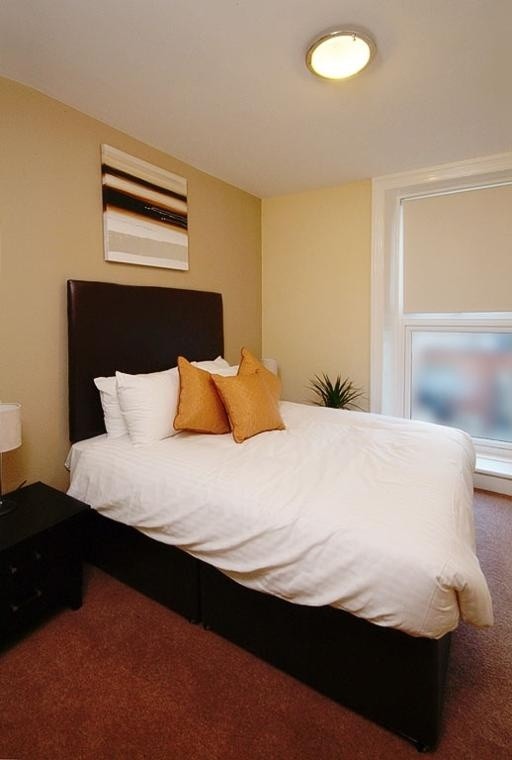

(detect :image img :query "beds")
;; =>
[65,278,493,754]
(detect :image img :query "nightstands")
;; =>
[0,481,91,655]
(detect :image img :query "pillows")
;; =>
[92,346,286,444]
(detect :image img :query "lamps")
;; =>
[304,25,377,83]
[0,403,23,515]
